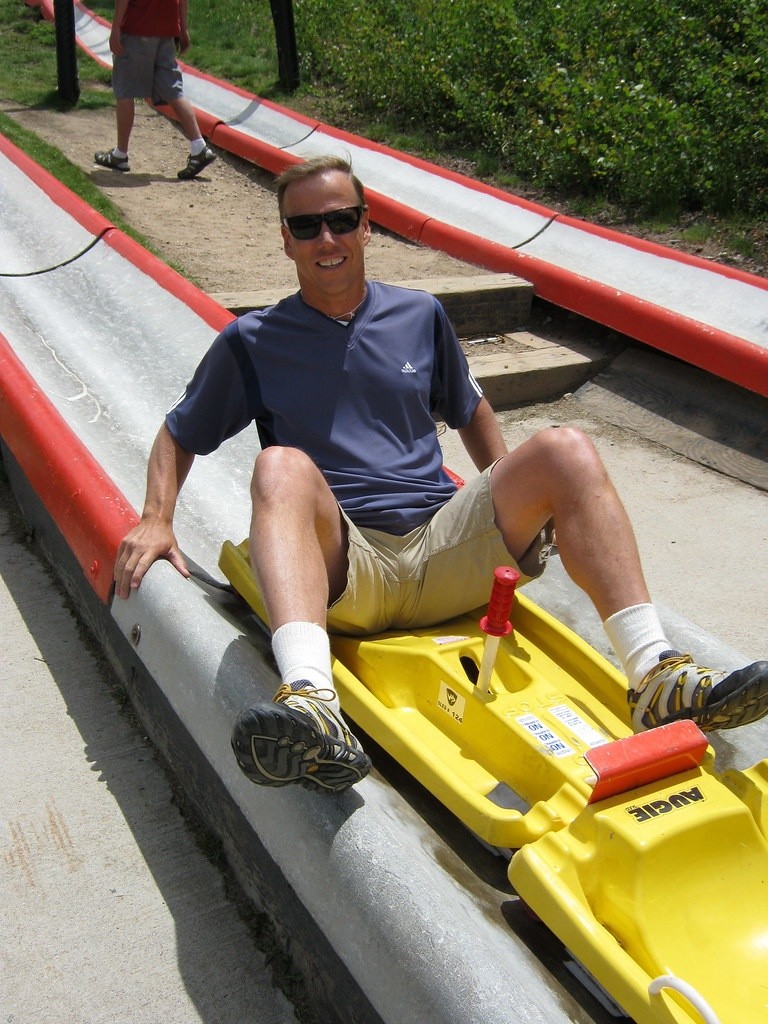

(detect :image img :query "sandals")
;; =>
[93,147,130,170]
[177,144,216,177]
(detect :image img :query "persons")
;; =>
[112,150,767,792]
[95,0,219,181]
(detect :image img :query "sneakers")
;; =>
[627,648,768,734]
[229,676,373,796]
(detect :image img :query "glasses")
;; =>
[280,200,369,239]
